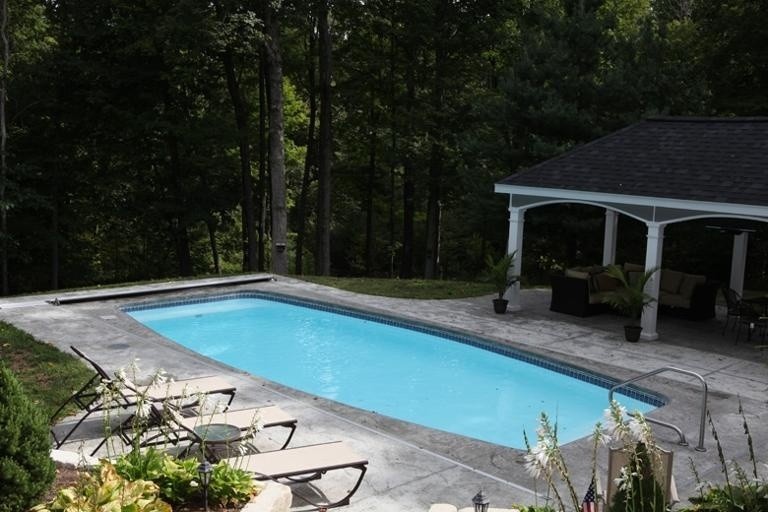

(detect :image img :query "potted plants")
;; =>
[601,264,662,342]
[484,248,527,313]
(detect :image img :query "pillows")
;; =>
[564,262,706,298]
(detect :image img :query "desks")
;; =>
[187,423,240,462]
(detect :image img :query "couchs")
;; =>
[547,270,721,321]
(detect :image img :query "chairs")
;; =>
[163,409,368,511]
[52,346,232,448]
[115,372,296,462]
[722,285,768,351]
